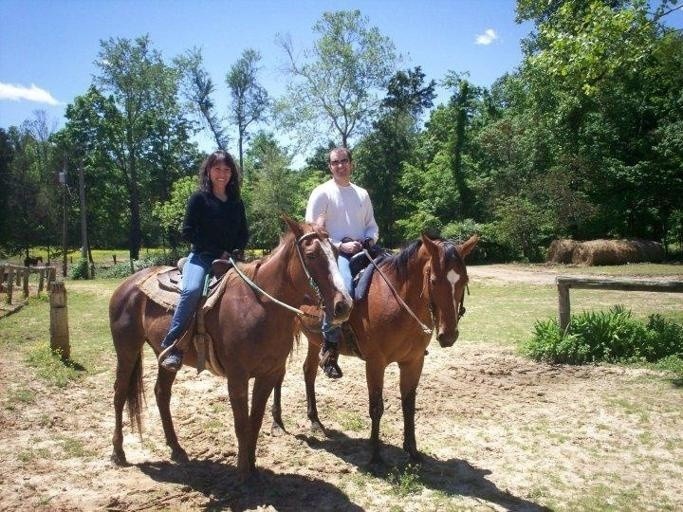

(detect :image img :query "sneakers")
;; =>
[162,350,182,372]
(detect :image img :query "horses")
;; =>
[270,229,480,466]
[108,203,355,495]
[24,255,44,268]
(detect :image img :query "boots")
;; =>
[322,340,343,378]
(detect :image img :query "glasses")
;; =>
[330,159,349,167]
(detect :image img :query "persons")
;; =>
[158,151,250,372]
[304,146,380,381]
